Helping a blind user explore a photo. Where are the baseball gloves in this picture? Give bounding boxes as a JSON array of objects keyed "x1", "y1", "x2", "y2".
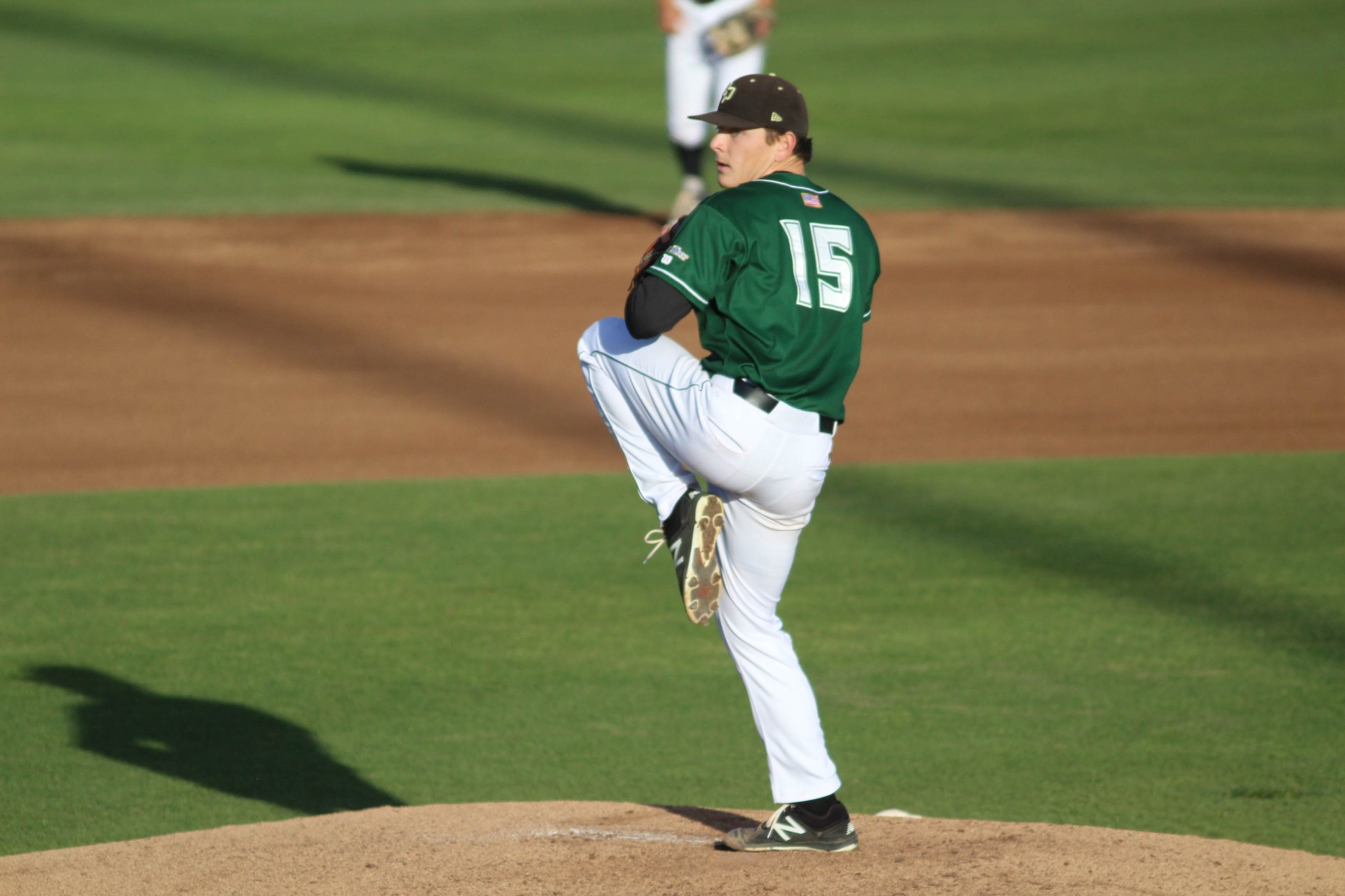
[{"x1": 704, "y1": 4, "x2": 777, "y2": 56}]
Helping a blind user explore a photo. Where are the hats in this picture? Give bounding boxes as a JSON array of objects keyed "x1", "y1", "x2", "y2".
[{"x1": 689, "y1": 72, "x2": 808, "y2": 140}]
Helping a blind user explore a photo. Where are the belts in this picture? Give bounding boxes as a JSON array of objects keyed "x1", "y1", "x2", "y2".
[{"x1": 732, "y1": 378, "x2": 834, "y2": 434}]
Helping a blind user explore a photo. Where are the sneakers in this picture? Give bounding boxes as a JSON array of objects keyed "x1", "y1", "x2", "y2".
[
  {"x1": 721, "y1": 802, "x2": 858, "y2": 853},
  {"x1": 663, "y1": 478, "x2": 724, "y2": 625}
]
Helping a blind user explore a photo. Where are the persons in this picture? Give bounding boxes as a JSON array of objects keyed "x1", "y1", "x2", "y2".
[
  {"x1": 660, "y1": 0, "x2": 773, "y2": 236},
  {"x1": 575, "y1": 73, "x2": 880, "y2": 854}
]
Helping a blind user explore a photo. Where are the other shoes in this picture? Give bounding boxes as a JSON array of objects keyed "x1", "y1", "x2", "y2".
[{"x1": 672, "y1": 176, "x2": 704, "y2": 217}]
